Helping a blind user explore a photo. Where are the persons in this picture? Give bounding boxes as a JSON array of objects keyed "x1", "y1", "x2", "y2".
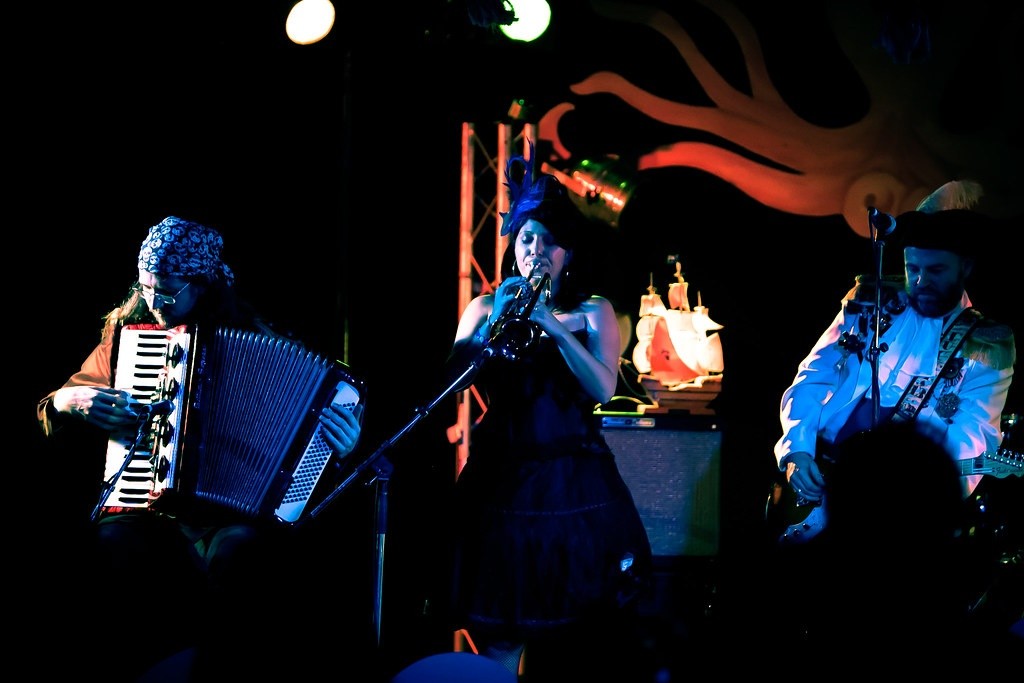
[
  {"x1": 32, "y1": 214, "x2": 364, "y2": 634},
  {"x1": 424, "y1": 176, "x2": 671, "y2": 683},
  {"x1": 668, "y1": 416, "x2": 1024, "y2": 683},
  {"x1": 772, "y1": 206, "x2": 1018, "y2": 548}
]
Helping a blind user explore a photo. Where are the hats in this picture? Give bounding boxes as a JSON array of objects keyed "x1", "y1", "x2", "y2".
[{"x1": 892, "y1": 178, "x2": 983, "y2": 263}]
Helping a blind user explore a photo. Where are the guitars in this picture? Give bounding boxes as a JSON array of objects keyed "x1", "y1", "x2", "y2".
[{"x1": 769, "y1": 427, "x2": 1024, "y2": 550}]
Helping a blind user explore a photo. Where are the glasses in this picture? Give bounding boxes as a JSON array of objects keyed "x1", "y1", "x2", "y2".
[{"x1": 132, "y1": 282, "x2": 191, "y2": 305}]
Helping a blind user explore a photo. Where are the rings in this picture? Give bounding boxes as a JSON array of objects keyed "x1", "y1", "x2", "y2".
[{"x1": 795, "y1": 489, "x2": 801, "y2": 495}]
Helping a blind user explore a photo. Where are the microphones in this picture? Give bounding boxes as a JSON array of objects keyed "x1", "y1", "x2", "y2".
[
  {"x1": 480, "y1": 327, "x2": 514, "y2": 359},
  {"x1": 867, "y1": 207, "x2": 897, "y2": 236},
  {"x1": 141, "y1": 400, "x2": 176, "y2": 415}
]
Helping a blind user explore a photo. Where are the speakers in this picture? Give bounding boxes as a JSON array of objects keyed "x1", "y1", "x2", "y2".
[{"x1": 595, "y1": 413, "x2": 730, "y2": 561}]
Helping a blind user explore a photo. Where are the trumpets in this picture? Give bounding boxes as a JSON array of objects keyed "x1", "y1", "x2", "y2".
[{"x1": 489, "y1": 258, "x2": 552, "y2": 362}]
